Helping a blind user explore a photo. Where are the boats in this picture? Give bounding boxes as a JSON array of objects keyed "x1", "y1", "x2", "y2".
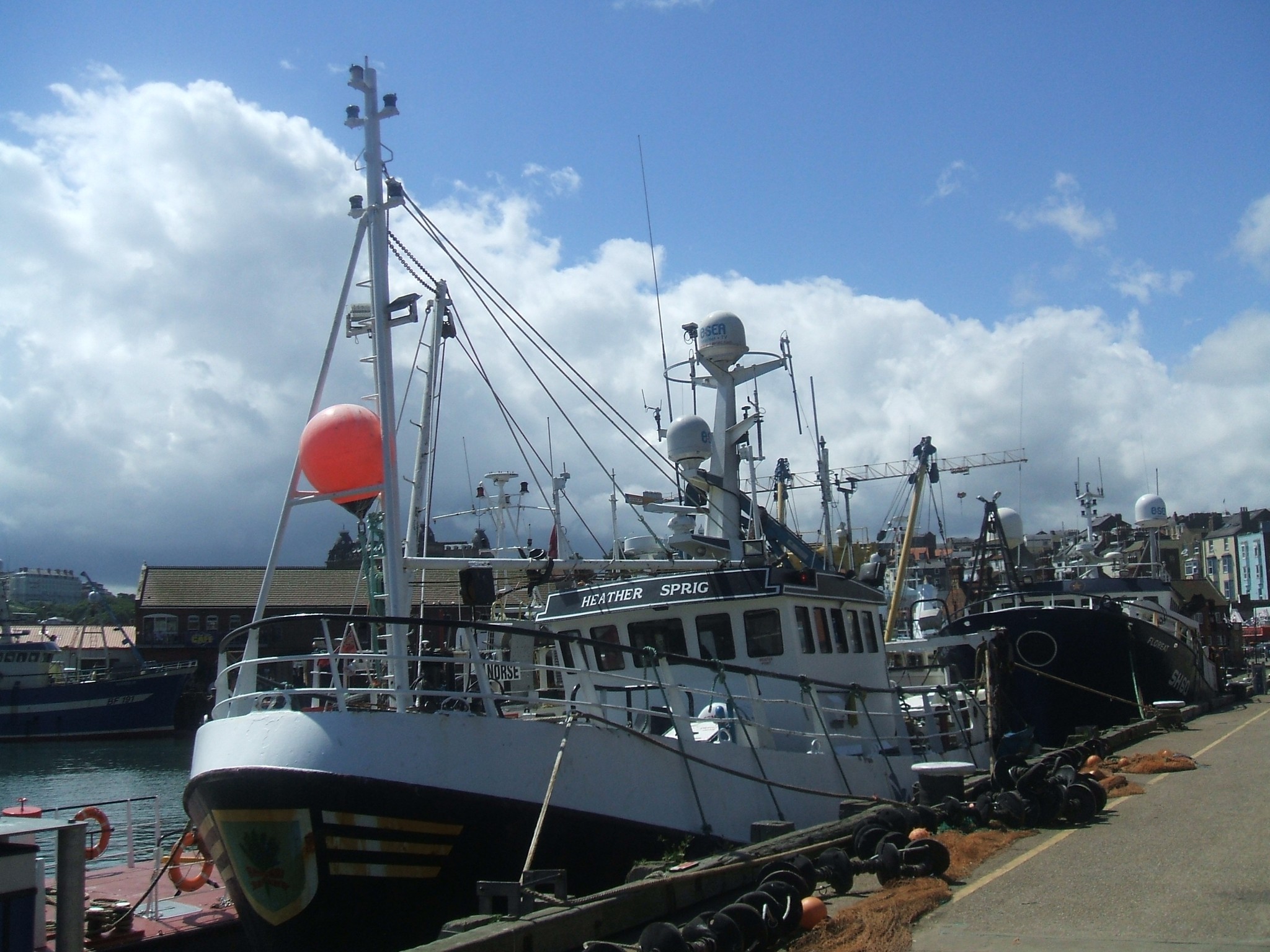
[
  {"x1": 180, "y1": 50, "x2": 1269, "y2": 952},
  {"x1": 0, "y1": 567, "x2": 200, "y2": 746}
]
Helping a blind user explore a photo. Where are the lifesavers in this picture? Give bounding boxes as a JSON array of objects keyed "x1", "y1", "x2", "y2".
[
  {"x1": 73, "y1": 806, "x2": 111, "y2": 859},
  {"x1": 170, "y1": 834, "x2": 213, "y2": 893}
]
